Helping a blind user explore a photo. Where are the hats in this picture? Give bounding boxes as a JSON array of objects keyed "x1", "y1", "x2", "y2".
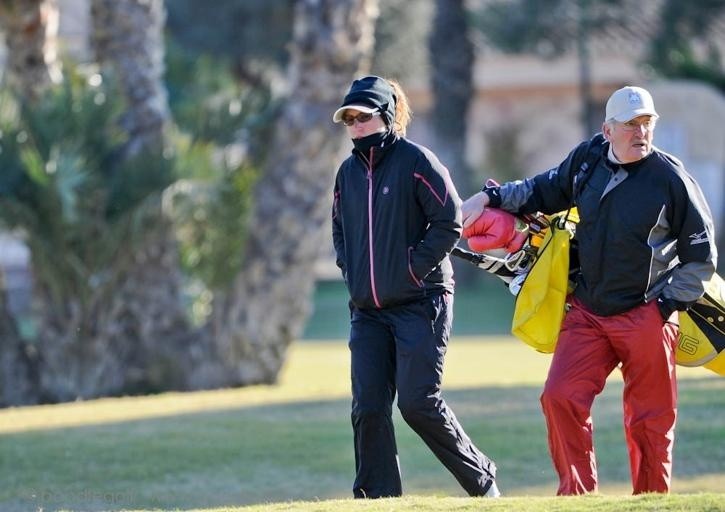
[
  {"x1": 333, "y1": 76, "x2": 395, "y2": 126},
  {"x1": 605, "y1": 86, "x2": 658, "y2": 124}
]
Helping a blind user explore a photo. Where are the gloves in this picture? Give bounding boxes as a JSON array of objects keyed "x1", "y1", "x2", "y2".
[{"x1": 461, "y1": 192, "x2": 490, "y2": 228}]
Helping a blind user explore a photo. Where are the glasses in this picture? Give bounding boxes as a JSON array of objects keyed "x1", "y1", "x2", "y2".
[
  {"x1": 342, "y1": 112, "x2": 381, "y2": 125},
  {"x1": 612, "y1": 121, "x2": 655, "y2": 130}
]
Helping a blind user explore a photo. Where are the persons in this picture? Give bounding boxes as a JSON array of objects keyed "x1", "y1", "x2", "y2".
[
  {"x1": 331, "y1": 76, "x2": 502, "y2": 501},
  {"x1": 462, "y1": 85, "x2": 720, "y2": 495}
]
